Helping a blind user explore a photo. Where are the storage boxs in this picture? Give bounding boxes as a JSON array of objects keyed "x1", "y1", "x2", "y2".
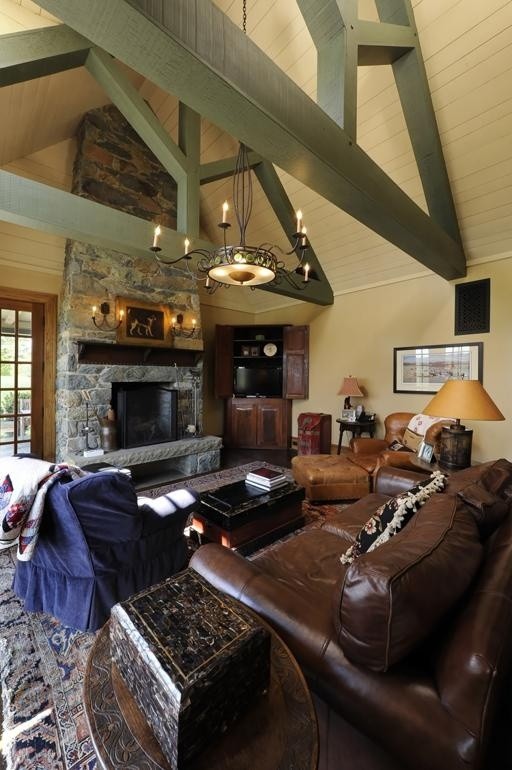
[{"x1": 110, "y1": 566, "x2": 272, "y2": 770}]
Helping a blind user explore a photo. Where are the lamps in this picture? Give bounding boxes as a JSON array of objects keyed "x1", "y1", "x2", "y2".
[
  {"x1": 171, "y1": 314, "x2": 196, "y2": 338},
  {"x1": 422, "y1": 380, "x2": 505, "y2": 470},
  {"x1": 91, "y1": 302, "x2": 124, "y2": 332},
  {"x1": 337, "y1": 375, "x2": 364, "y2": 409},
  {"x1": 150, "y1": 0, "x2": 311, "y2": 295}
]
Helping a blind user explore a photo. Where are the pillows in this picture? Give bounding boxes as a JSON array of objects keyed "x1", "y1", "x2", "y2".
[{"x1": 333, "y1": 459, "x2": 512, "y2": 675}]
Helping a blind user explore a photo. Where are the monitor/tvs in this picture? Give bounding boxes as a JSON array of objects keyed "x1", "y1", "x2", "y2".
[{"x1": 233, "y1": 363, "x2": 282, "y2": 398}]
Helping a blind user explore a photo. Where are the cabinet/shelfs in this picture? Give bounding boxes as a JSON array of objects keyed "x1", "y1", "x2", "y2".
[{"x1": 213, "y1": 323, "x2": 309, "y2": 450}]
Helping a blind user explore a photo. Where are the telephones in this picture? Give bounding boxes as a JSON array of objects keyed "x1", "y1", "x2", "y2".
[{"x1": 358, "y1": 411, "x2": 376, "y2": 422}]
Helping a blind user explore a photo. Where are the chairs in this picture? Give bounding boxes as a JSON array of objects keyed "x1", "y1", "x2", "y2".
[
  {"x1": 347, "y1": 412, "x2": 457, "y2": 490},
  {"x1": 11, "y1": 453, "x2": 201, "y2": 632}
]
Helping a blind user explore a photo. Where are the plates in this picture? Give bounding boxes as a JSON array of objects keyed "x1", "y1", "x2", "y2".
[{"x1": 263, "y1": 344, "x2": 278, "y2": 358}]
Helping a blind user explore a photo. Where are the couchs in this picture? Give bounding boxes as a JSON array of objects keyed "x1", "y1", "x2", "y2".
[{"x1": 188, "y1": 459, "x2": 512, "y2": 770}]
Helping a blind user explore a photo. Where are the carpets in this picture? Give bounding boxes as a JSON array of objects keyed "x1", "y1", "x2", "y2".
[{"x1": 0, "y1": 460, "x2": 360, "y2": 770}]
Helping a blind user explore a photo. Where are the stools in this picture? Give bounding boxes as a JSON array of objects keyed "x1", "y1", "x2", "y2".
[{"x1": 291, "y1": 454, "x2": 369, "y2": 510}]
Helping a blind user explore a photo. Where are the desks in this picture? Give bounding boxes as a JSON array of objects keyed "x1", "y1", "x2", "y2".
[
  {"x1": 84, "y1": 590, "x2": 320, "y2": 770},
  {"x1": 409, "y1": 456, "x2": 482, "y2": 477},
  {"x1": 336, "y1": 418, "x2": 377, "y2": 455}
]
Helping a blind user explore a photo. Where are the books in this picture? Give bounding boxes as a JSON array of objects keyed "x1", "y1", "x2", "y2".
[{"x1": 244, "y1": 467, "x2": 289, "y2": 492}]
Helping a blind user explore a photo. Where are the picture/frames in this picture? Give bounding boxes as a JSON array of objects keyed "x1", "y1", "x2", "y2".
[
  {"x1": 341, "y1": 409, "x2": 355, "y2": 420},
  {"x1": 418, "y1": 441, "x2": 436, "y2": 464},
  {"x1": 394, "y1": 342, "x2": 483, "y2": 395}
]
